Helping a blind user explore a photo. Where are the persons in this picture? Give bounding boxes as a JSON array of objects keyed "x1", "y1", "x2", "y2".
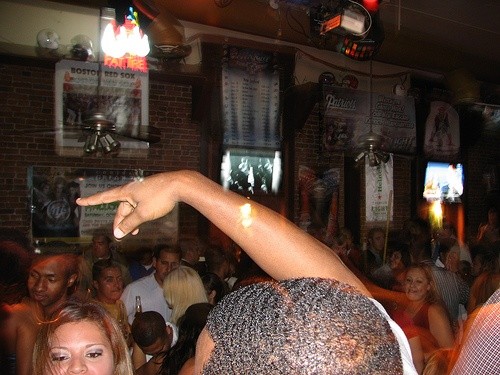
[
  {"x1": 0, "y1": 210, "x2": 500, "y2": 375},
  {"x1": 37, "y1": 178, "x2": 81, "y2": 222},
  {"x1": 76, "y1": 169, "x2": 419, "y2": 375},
  {"x1": 228, "y1": 159, "x2": 273, "y2": 194}
]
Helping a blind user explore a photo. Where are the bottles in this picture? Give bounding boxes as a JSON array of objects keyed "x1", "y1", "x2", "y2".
[{"x1": 136, "y1": 296, "x2": 142, "y2": 312}]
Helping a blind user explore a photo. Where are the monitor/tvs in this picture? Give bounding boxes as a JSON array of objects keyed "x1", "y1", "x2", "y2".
[
  {"x1": 420, "y1": 159, "x2": 467, "y2": 204},
  {"x1": 219, "y1": 146, "x2": 284, "y2": 197}
]
